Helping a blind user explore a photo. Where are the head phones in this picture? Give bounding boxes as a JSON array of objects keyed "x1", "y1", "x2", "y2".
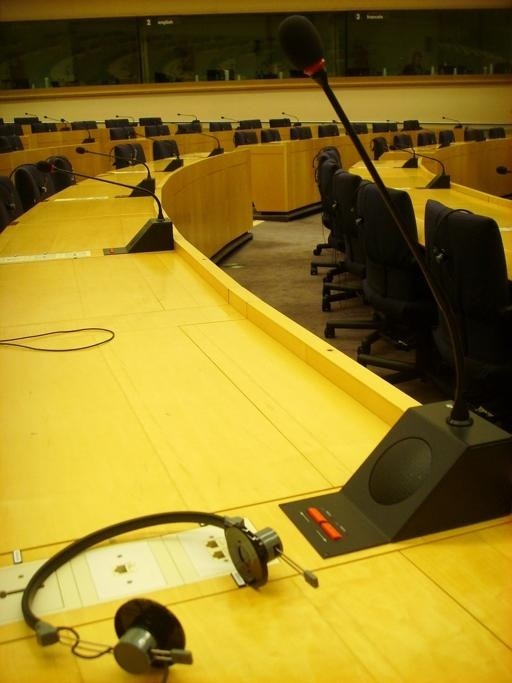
[
  {"x1": 313, "y1": 148, "x2": 345, "y2": 220},
  {"x1": 424, "y1": 208, "x2": 473, "y2": 277},
  {"x1": 22, "y1": 511, "x2": 321, "y2": 676},
  {"x1": 5, "y1": 164, "x2": 48, "y2": 210},
  {"x1": 349, "y1": 182, "x2": 375, "y2": 240}
]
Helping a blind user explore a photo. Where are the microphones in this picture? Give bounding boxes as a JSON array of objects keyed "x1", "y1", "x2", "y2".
[
  {"x1": 176, "y1": 112, "x2": 200, "y2": 126},
  {"x1": 333, "y1": 119, "x2": 338, "y2": 124},
  {"x1": 442, "y1": 116, "x2": 462, "y2": 129},
  {"x1": 130, "y1": 130, "x2": 160, "y2": 147},
  {"x1": 36, "y1": 161, "x2": 175, "y2": 255},
  {"x1": 115, "y1": 114, "x2": 137, "y2": 126},
  {"x1": 25, "y1": 112, "x2": 42, "y2": 123},
  {"x1": 44, "y1": 116, "x2": 70, "y2": 131},
  {"x1": 222, "y1": 116, "x2": 241, "y2": 130},
  {"x1": 61, "y1": 118, "x2": 95, "y2": 144},
  {"x1": 197, "y1": 129, "x2": 224, "y2": 157},
  {"x1": 389, "y1": 145, "x2": 450, "y2": 189},
  {"x1": 272, "y1": 15, "x2": 512, "y2": 562},
  {"x1": 282, "y1": 113, "x2": 299, "y2": 122},
  {"x1": 496, "y1": 166, "x2": 512, "y2": 175},
  {"x1": 75, "y1": 147, "x2": 156, "y2": 198}
]
